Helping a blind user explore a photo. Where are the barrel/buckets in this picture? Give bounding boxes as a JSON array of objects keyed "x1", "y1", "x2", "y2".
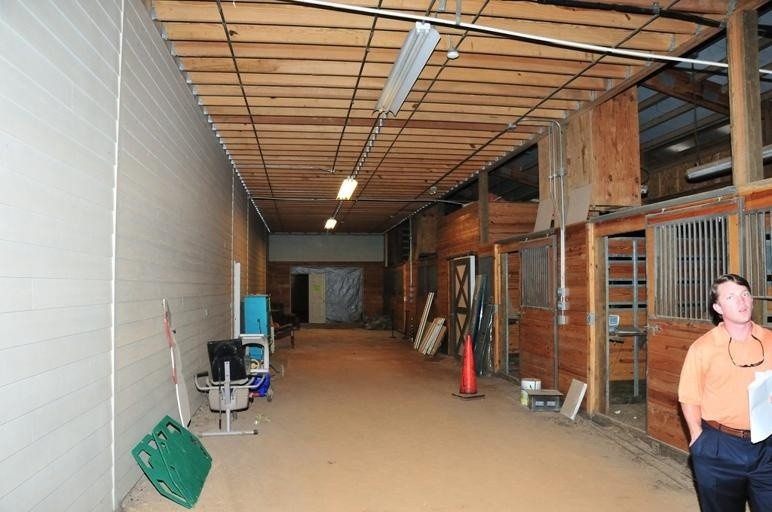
[{"x1": 521, "y1": 378, "x2": 542, "y2": 406}]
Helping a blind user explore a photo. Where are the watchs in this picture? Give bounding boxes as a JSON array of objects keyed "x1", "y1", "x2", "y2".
[{"x1": 672, "y1": 271, "x2": 772, "y2": 511}]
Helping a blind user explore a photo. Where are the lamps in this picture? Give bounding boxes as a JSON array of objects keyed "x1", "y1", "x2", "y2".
[
  {"x1": 336, "y1": 179, "x2": 358, "y2": 201},
  {"x1": 324, "y1": 218, "x2": 337, "y2": 230},
  {"x1": 684, "y1": 53, "x2": 772, "y2": 180},
  {"x1": 371, "y1": 23, "x2": 441, "y2": 116}
]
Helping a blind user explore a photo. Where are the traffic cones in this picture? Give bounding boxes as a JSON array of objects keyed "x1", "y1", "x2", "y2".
[{"x1": 451, "y1": 333, "x2": 484, "y2": 399}]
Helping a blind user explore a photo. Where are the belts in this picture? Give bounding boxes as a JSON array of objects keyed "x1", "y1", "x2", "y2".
[{"x1": 704, "y1": 419, "x2": 751, "y2": 441}]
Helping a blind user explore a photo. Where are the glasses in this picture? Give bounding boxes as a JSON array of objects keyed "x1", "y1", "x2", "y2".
[{"x1": 721, "y1": 334, "x2": 768, "y2": 372}]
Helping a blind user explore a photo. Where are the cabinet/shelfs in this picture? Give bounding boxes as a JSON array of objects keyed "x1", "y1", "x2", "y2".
[{"x1": 244, "y1": 296, "x2": 269, "y2": 362}]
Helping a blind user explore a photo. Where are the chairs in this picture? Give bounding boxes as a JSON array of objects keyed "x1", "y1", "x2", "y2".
[{"x1": 270, "y1": 303, "x2": 300, "y2": 349}]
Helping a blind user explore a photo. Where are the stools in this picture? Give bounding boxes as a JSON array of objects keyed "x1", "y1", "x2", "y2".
[{"x1": 529, "y1": 390, "x2": 563, "y2": 411}]
